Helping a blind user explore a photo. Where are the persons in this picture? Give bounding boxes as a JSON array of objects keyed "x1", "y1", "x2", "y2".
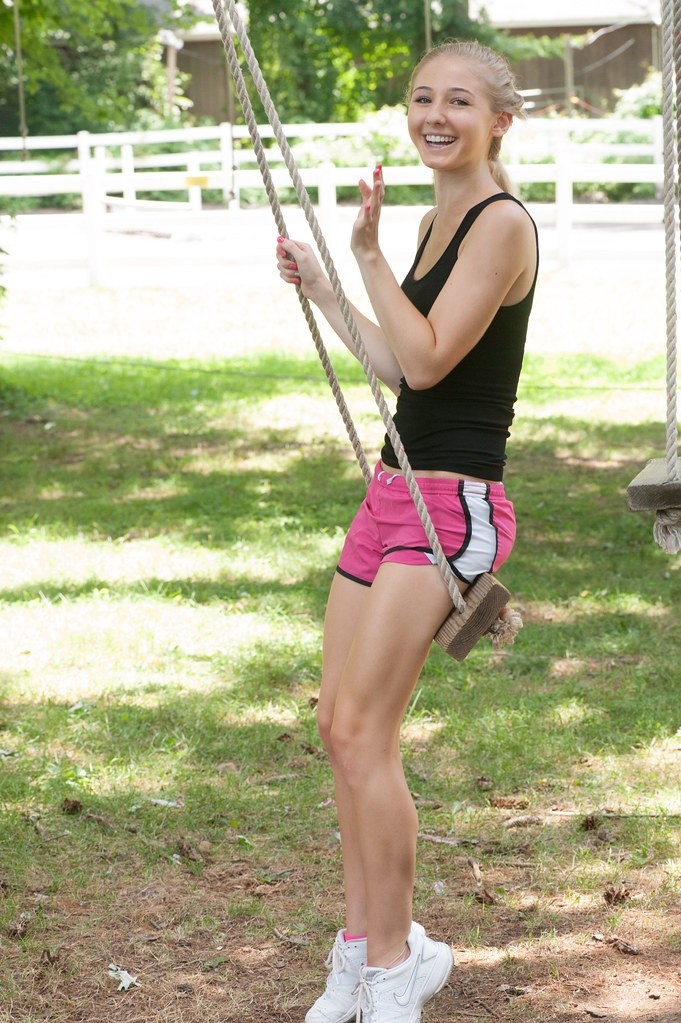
[{"x1": 277, "y1": 43, "x2": 538, "y2": 1023}]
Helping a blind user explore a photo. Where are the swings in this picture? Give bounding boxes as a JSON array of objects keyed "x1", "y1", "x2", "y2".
[
  {"x1": 214, "y1": 1, "x2": 513, "y2": 665},
  {"x1": 622, "y1": 2, "x2": 680, "y2": 514}
]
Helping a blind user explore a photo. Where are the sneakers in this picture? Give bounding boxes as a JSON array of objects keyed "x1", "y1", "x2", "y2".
[
  {"x1": 305, "y1": 922, "x2": 429, "y2": 1023},
  {"x1": 351, "y1": 929, "x2": 456, "y2": 1023}
]
